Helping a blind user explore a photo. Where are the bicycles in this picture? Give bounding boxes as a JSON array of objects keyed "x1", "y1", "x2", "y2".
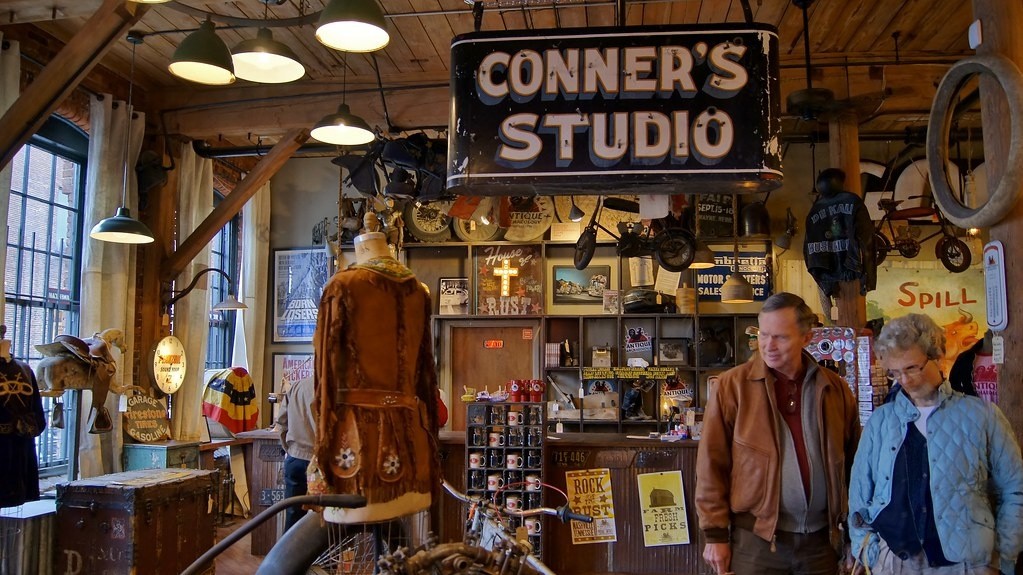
[
  {"x1": 574, "y1": 194, "x2": 698, "y2": 272},
  {"x1": 870, "y1": 192, "x2": 972, "y2": 273},
  {"x1": 176, "y1": 478, "x2": 594, "y2": 575}
]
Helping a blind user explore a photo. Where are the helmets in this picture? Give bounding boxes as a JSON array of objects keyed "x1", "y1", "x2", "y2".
[{"x1": 816, "y1": 167, "x2": 846, "y2": 199}]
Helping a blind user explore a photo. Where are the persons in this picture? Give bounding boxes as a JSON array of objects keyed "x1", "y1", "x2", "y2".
[
  {"x1": 846, "y1": 313, "x2": 1023, "y2": 575},
  {"x1": 694, "y1": 291, "x2": 861, "y2": 575},
  {"x1": 949, "y1": 329, "x2": 998, "y2": 407},
  {"x1": 0, "y1": 338, "x2": 46, "y2": 507},
  {"x1": 275, "y1": 372, "x2": 317, "y2": 535},
  {"x1": 306, "y1": 232, "x2": 444, "y2": 525}
]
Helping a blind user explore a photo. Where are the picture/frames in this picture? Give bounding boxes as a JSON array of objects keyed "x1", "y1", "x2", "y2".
[
  {"x1": 438, "y1": 277, "x2": 468, "y2": 315},
  {"x1": 651, "y1": 337, "x2": 691, "y2": 367},
  {"x1": 553, "y1": 265, "x2": 611, "y2": 305},
  {"x1": 270, "y1": 247, "x2": 334, "y2": 428}
]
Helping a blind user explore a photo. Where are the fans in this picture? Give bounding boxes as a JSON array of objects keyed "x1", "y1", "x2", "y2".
[{"x1": 779, "y1": 0, "x2": 913, "y2": 122}]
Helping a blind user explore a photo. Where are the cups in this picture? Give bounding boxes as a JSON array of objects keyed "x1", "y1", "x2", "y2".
[
  {"x1": 489, "y1": 432, "x2": 504, "y2": 447},
  {"x1": 506, "y1": 497, "x2": 522, "y2": 511},
  {"x1": 488, "y1": 476, "x2": 504, "y2": 490},
  {"x1": 525, "y1": 517, "x2": 542, "y2": 534},
  {"x1": 675, "y1": 288, "x2": 695, "y2": 314},
  {"x1": 469, "y1": 453, "x2": 485, "y2": 468},
  {"x1": 525, "y1": 476, "x2": 541, "y2": 490},
  {"x1": 507, "y1": 411, "x2": 523, "y2": 426},
  {"x1": 474, "y1": 434, "x2": 481, "y2": 446},
  {"x1": 506, "y1": 454, "x2": 522, "y2": 469}
]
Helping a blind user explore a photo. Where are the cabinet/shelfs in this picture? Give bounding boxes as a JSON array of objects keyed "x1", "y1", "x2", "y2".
[
  {"x1": 340, "y1": 238, "x2": 772, "y2": 564},
  {"x1": 121, "y1": 441, "x2": 199, "y2": 473}
]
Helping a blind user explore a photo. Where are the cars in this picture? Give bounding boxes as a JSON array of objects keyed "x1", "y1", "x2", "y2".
[{"x1": 441, "y1": 288, "x2": 469, "y2": 305}]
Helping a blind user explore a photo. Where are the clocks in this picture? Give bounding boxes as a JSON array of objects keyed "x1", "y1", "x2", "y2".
[{"x1": 147, "y1": 334, "x2": 187, "y2": 395}]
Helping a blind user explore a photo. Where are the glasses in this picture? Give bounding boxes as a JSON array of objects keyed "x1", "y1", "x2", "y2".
[
  {"x1": 786, "y1": 379, "x2": 797, "y2": 414},
  {"x1": 886, "y1": 356, "x2": 929, "y2": 381}
]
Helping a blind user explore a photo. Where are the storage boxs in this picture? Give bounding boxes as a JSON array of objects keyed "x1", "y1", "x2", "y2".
[{"x1": 54, "y1": 468, "x2": 219, "y2": 575}]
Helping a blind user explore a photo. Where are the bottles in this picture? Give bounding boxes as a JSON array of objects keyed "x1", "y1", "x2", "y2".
[
  {"x1": 509, "y1": 429, "x2": 517, "y2": 446},
  {"x1": 528, "y1": 451, "x2": 534, "y2": 468},
  {"x1": 530, "y1": 408, "x2": 536, "y2": 425},
  {"x1": 472, "y1": 471, "x2": 478, "y2": 489},
  {"x1": 508, "y1": 472, "x2": 516, "y2": 489},
  {"x1": 489, "y1": 493, "x2": 536, "y2": 510},
  {"x1": 528, "y1": 429, "x2": 536, "y2": 446},
  {"x1": 508, "y1": 514, "x2": 515, "y2": 532},
  {"x1": 491, "y1": 450, "x2": 497, "y2": 467},
  {"x1": 491, "y1": 407, "x2": 499, "y2": 424},
  {"x1": 529, "y1": 538, "x2": 534, "y2": 554},
  {"x1": 505, "y1": 380, "x2": 546, "y2": 402}
]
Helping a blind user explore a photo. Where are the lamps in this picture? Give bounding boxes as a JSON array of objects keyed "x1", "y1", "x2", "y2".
[
  {"x1": 230, "y1": 0, "x2": 305, "y2": 83},
  {"x1": 310, "y1": 52, "x2": 375, "y2": 145},
  {"x1": 687, "y1": 193, "x2": 716, "y2": 269},
  {"x1": 165, "y1": 13, "x2": 235, "y2": 85},
  {"x1": 89, "y1": 28, "x2": 154, "y2": 244},
  {"x1": 720, "y1": 194, "x2": 754, "y2": 304},
  {"x1": 773, "y1": 207, "x2": 797, "y2": 250},
  {"x1": 568, "y1": 194, "x2": 585, "y2": 222},
  {"x1": 162, "y1": 268, "x2": 249, "y2": 315},
  {"x1": 314, "y1": 0, "x2": 391, "y2": 53}
]
